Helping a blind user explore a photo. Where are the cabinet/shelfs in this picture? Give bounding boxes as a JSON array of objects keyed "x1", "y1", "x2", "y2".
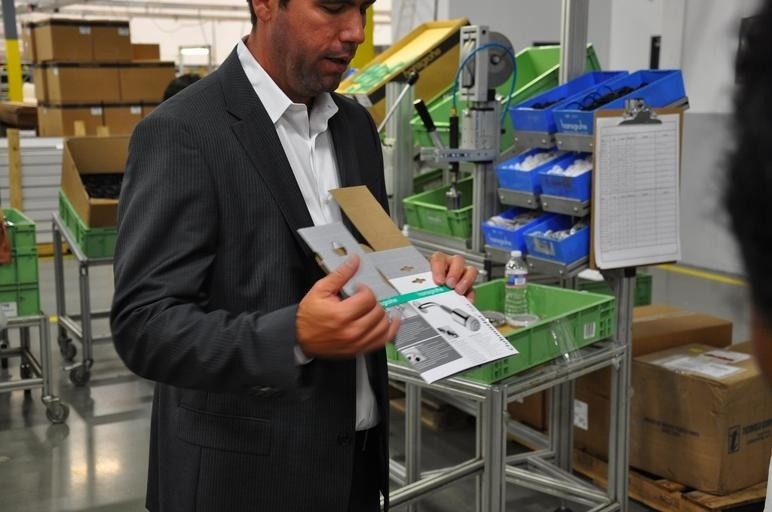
[{"x1": 340, "y1": 47, "x2": 637, "y2": 511}]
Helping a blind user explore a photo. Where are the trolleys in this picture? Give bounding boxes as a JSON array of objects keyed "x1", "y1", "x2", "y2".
[
  {"x1": 1, "y1": 313, "x2": 68, "y2": 424},
  {"x1": 51, "y1": 214, "x2": 115, "y2": 388}
]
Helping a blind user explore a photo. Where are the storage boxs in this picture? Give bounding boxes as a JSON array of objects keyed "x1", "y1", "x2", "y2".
[
  {"x1": 544, "y1": 303, "x2": 772, "y2": 495},
  {"x1": 20, "y1": 15, "x2": 180, "y2": 228}
]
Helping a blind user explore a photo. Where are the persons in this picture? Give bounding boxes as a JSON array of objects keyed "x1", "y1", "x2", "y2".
[
  {"x1": 158, "y1": 70, "x2": 201, "y2": 101},
  {"x1": 696, "y1": 1, "x2": 771, "y2": 387},
  {"x1": 106, "y1": 2, "x2": 482, "y2": 512}
]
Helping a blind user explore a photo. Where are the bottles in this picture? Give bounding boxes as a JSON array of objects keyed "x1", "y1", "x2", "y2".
[{"x1": 506, "y1": 252, "x2": 529, "y2": 322}]
